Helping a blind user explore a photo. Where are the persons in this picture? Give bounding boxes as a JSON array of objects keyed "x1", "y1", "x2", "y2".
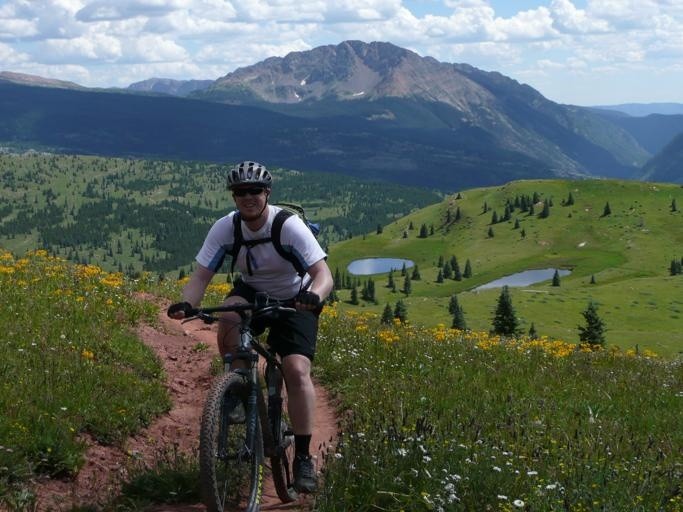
[{"x1": 164, "y1": 159, "x2": 336, "y2": 496}]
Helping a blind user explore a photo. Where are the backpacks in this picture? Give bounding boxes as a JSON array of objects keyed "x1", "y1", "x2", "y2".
[{"x1": 227, "y1": 202, "x2": 320, "y2": 288}]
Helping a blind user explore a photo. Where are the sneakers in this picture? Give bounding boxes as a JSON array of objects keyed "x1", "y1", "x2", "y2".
[
  {"x1": 292, "y1": 455, "x2": 318, "y2": 494},
  {"x1": 227, "y1": 392, "x2": 248, "y2": 424}
]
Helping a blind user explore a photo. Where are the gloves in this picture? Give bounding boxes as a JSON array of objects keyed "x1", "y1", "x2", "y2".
[
  {"x1": 167, "y1": 303, "x2": 194, "y2": 317},
  {"x1": 294, "y1": 289, "x2": 320, "y2": 305}
]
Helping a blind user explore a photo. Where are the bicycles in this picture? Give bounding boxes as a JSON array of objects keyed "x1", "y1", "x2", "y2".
[{"x1": 170, "y1": 294, "x2": 297, "y2": 512}]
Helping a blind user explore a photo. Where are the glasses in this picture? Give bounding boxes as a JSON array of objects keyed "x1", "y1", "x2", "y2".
[{"x1": 233, "y1": 188, "x2": 264, "y2": 197}]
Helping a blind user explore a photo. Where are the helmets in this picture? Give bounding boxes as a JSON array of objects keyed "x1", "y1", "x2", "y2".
[{"x1": 227, "y1": 161, "x2": 273, "y2": 188}]
